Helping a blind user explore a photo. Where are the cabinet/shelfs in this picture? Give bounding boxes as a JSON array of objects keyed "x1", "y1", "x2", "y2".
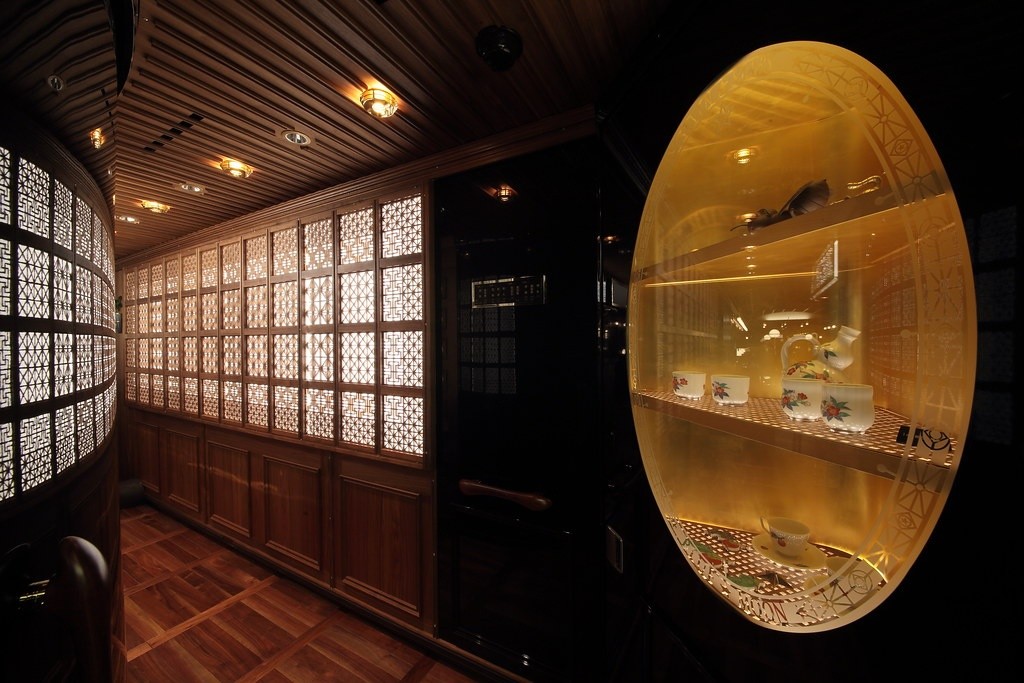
[{"x1": 628, "y1": 165, "x2": 960, "y2": 630}]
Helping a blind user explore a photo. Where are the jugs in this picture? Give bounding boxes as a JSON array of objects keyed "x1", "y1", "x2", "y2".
[{"x1": 780, "y1": 325, "x2": 861, "y2": 384}]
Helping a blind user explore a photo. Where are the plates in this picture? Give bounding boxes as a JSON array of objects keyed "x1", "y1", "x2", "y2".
[
  {"x1": 826, "y1": 557, "x2": 858, "y2": 581},
  {"x1": 752, "y1": 533, "x2": 827, "y2": 569}
]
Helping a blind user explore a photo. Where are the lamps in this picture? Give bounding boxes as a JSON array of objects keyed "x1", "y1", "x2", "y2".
[
  {"x1": 141, "y1": 199, "x2": 170, "y2": 215},
  {"x1": 358, "y1": 85, "x2": 401, "y2": 124},
  {"x1": 215, "y1": 154, "x2": 257, "y2": 183},
  {"x1": 89, "y1": 127, "x2": 104, "y2": 150},
  {"x1": 492, "y1": 183, "x2": 522, "y2": 206}
]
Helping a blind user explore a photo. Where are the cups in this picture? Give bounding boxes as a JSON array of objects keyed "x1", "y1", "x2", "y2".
[
  {"x1": 671, "y1": 370, "x2": 707, "y2": 401},
  {"x1": 760, "y1": 515, "x2": 810, "y2": 557},
  {"x1": 780, "y1": 378, "x2": 826, "y2": 422},
  {"x1": 821, "y1": 382, "x2": 876, "y2": 435},
  {"x1": 710, "y1": 374, "x2": 750, "y2": 407}
]
